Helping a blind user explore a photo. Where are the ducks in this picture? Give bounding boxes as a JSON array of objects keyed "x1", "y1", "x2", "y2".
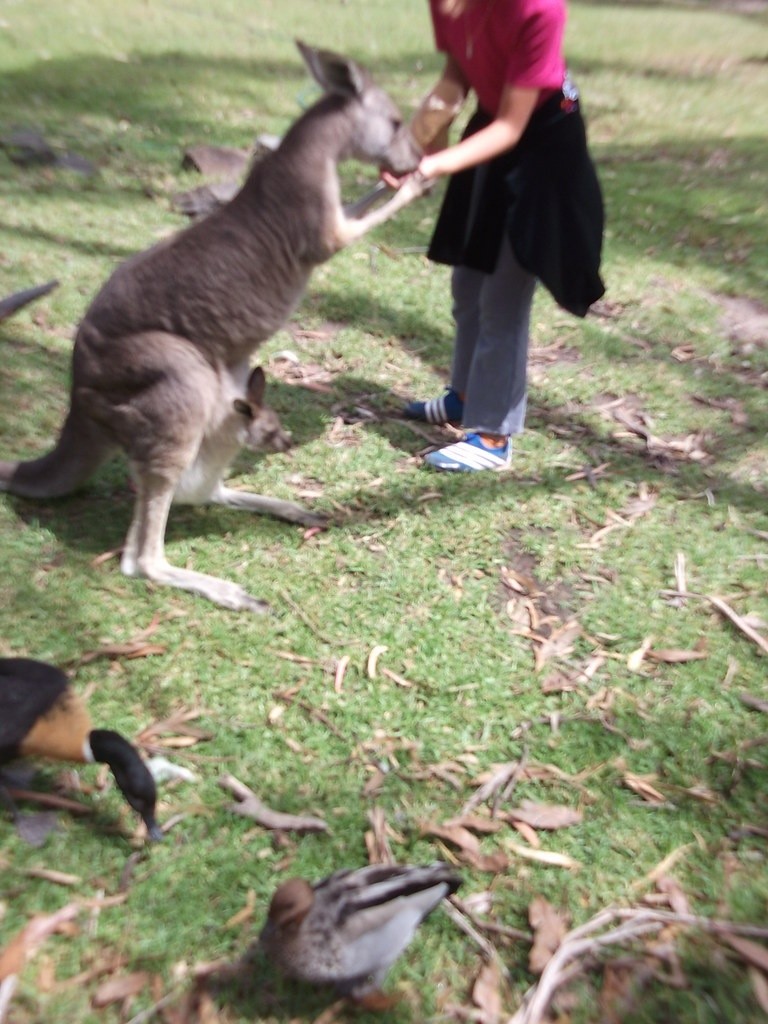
[
  {"x1": 1, "y1": 653, "x2": 172, "y2": 847},
  {"x1": 250, "y1": 857, "x2": 468, "y2": 1015}
]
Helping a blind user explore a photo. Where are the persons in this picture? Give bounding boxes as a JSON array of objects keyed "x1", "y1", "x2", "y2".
[{"x1": 382, "y1": 0, "x2": 607, "y2": 471}]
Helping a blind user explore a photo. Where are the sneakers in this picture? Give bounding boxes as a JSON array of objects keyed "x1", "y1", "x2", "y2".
[
  {"x1": 425, "y1": 433, "x2": 511, "y2": 471},
  {"x1": 405, "y1": 390, "x2": 465, "y2": 424}
]
